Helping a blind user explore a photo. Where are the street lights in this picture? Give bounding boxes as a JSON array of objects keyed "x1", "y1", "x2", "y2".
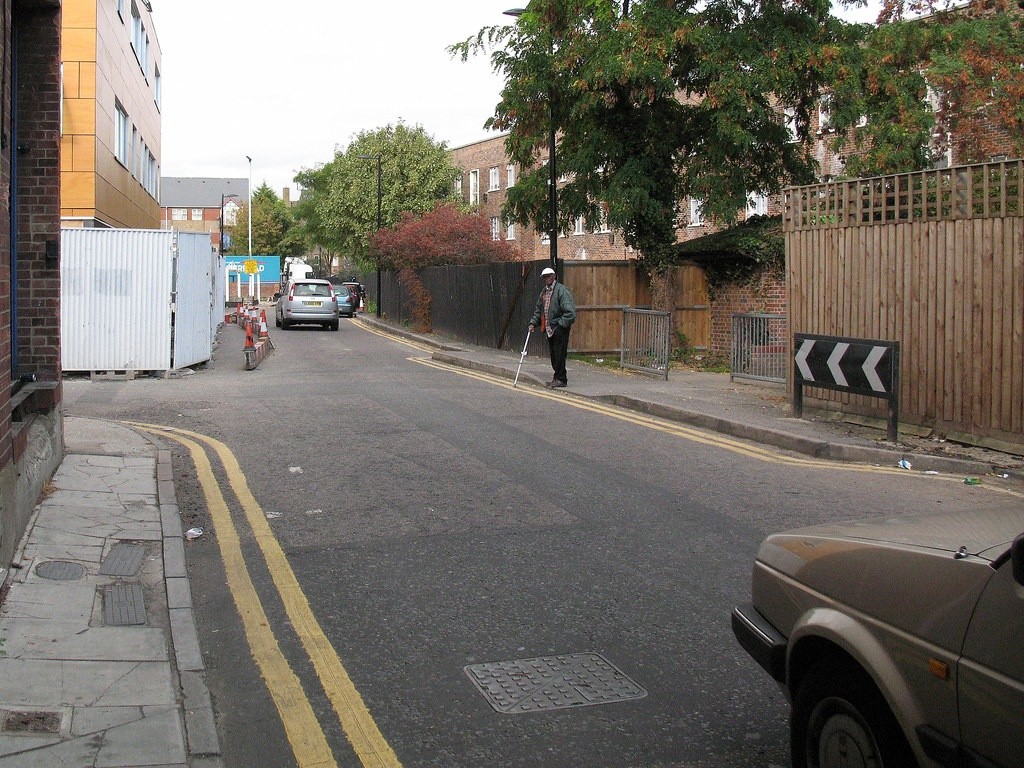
[
  {"x1": 219, "y1": 193, "x2": 239, "y2": 257},
  {"x1": 503, "y1": 7, "x2": 558, "y2": 288},
  {"x1": 243, "y1": 154, "x2": 258, "y2": 300},
  {"x1": 358, "y1": 153, "x2": 383, "y2": 319}
]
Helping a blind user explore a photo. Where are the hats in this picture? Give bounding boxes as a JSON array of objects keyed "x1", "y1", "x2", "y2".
[{"x1": 540, "y1": 268, "x2": 555, "y2": 277}]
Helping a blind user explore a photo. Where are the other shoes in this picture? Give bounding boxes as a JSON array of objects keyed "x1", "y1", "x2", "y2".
[{"x1": 547, "y1": 378, "x2": 566, "y2": 386}]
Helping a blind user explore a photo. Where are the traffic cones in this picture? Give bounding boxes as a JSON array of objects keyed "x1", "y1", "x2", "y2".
[
  {"x1": 358, "y1": 297, "x2": 364, "y2": 311},
  {"x1": 243, "y1": 321, "x2": 257, "y2": 352},
  {"x1": 238, "y1": 302, "x2": 268, "y2": 338}
]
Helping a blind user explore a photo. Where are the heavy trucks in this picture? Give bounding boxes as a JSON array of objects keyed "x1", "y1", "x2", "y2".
[{"x1": 225, "y1": 255, "x2": 313, "y2": 303}]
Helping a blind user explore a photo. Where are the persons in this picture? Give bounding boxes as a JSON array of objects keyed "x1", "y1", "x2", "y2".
[{"x1": 528, "y1": 267, "x2": 576, "y2": 388}]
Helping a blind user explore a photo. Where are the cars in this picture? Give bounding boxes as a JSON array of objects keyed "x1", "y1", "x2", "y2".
[
  {"x1": 273, "y1": 278, "x2": 340, "y2": 331},
  {"x1": 322, "y1": 282, "x2": 362, "y2": 318},
  {"x1": 730, "y1": 500, "x2": 1023, "y2": 768}
]
